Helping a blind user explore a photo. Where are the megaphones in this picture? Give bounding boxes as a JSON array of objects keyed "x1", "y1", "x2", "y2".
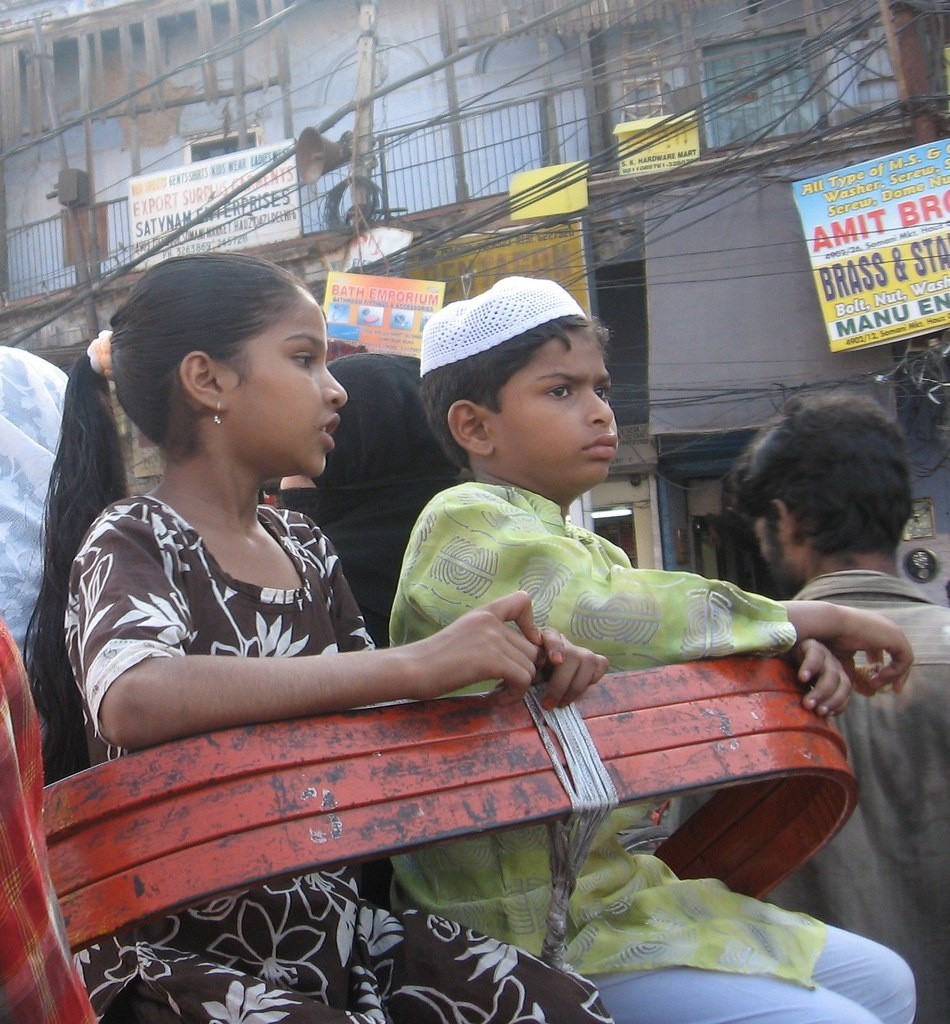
[{"x1": 295, "y1": 126, "x2": 351, "y2": 185}]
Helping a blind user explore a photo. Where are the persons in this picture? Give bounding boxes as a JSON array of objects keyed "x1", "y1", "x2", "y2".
[
  {"x1": 20, "y1": 253, "x2": 613, "y2": 1023},
  {"x1": 731, "y1": 388, "x2": 950, "y2": 1024},
  {"x1": 280, "y1": 274, "x2": 920, "y2": 1023}
]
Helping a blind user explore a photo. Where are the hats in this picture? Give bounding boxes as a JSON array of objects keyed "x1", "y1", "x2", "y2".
[{"x1": 417, "y1": 277, "x2": 593, "y2": 377}]
